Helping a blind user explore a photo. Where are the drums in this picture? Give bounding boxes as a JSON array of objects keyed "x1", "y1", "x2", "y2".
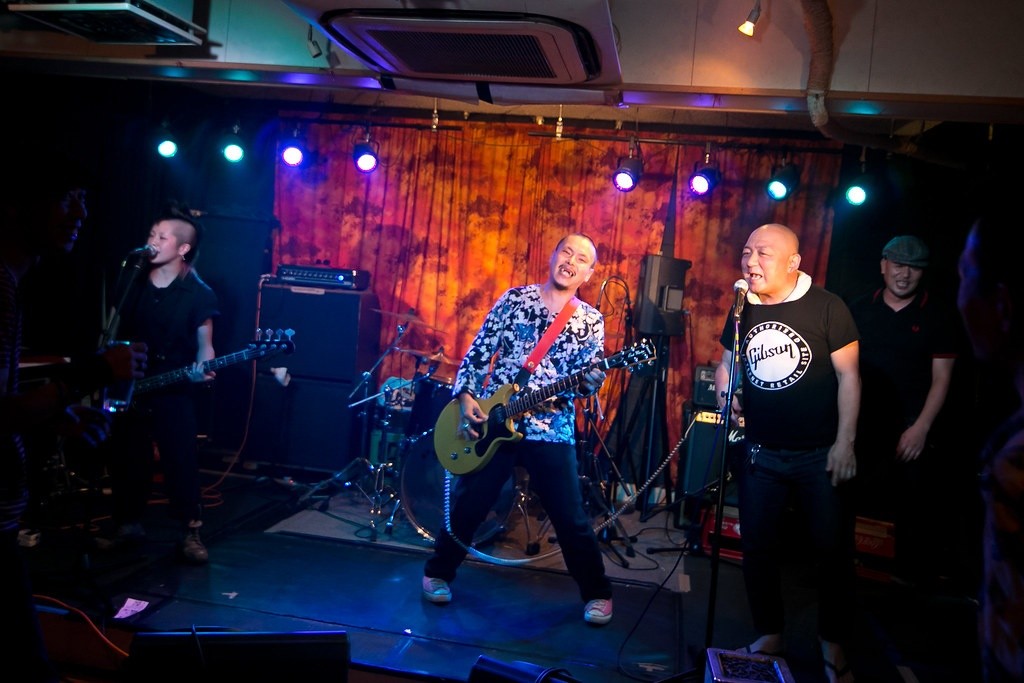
[
  {"x1": 403, "y1": 373, "x2": 456, "y2": 443},
  {"x1": 398, "y1": 428, "x2": 519, "y2": 547}
]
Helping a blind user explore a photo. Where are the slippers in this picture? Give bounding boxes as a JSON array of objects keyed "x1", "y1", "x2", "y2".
[
  {"x1": 734, "y1": 643, "x2": 792, "y2": 658},
  {"x1": 822, "y1": 657, "x2": 855, "y2": 683}
]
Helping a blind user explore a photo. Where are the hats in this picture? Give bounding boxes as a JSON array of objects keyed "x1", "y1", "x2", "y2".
[{"x1": 882, "y1": 236, "x2": 930, "y2": 267}]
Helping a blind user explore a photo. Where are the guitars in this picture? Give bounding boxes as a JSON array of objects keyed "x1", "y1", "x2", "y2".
[
  {"x1": 434, "y1": 338, "x2": 659, "y2": 476},
  {"x1": 82, "y1": 326, "x2": 298, "y2": 416}
]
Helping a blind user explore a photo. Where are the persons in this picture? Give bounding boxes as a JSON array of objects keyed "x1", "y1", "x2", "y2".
[
  {"x1": 110, "y1": 218, "x2": 221, "y2": 564},
  {"x1": 422, "y1": 233, "x2": 613, "y2": 624},
  {"x1": 958, "y1": 218, "x2": 1024, "y2": 683},
  {"x1": 0, "y1": 171, "x2": 89, "y2": 683},
  {"x1": 714, "y1": 222, "x2": 862, "y2": 683},
  {"x1": 874, "y1": 236, "x2": 974, "y2": 566}
]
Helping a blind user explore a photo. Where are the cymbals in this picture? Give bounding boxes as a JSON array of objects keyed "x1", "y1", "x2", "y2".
[
  {"x1": 402, "y1": 348, "x2": 463, "y2": 366},
  {"x1": 370, "y1": 307, "x2": 449, "y2": 335}
]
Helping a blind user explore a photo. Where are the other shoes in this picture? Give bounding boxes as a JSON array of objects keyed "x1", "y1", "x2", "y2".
[
  {"x1": 583, "y1": 598, "x2": 613, "y2": 624},
  {"x1": 422, "y1": 575, "x2": 452, "y2": 601},
  {"x1": 182, "y1": 519, "x2": 210, "y2": 562}
]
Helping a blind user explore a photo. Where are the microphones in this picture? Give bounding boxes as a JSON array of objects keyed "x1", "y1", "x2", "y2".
[
  {"x1": 131, "y1": 245, "x2": 158, "y2": 258},
  {"x1": 733, "y1": 278, "x2": 749, "y2": 318}
]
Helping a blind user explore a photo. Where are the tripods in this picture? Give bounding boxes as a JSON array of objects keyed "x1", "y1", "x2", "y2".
[
  {"x1": 647, "y1": 317, "x2": 745, "y2": 569},
  {"x1": 532, "y1": 388, "x2": 639, "y2": 567},
  {"x1": 287, "y1": 321, "x2": 439, "y2": 540}
]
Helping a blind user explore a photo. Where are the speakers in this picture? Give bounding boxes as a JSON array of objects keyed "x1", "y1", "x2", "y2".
[
  {"x1": 678, "y1": 408, "x2": 746, "y2": 500},
  {"x1": 254, "y1": 282, "x2": 381, "y2": 382},
  {"x1": 119, "y1": 629, "x2": 352, "y2": 683},
  {"x1": 632, "y1": 254, "x2": 692, "y2": 335},
  {"x1": 174, "y1": 209, "x2": 275, "y2": 456},
  {"x1": 248, "y1": 374, "x2": 377, "y2": 474}
]
